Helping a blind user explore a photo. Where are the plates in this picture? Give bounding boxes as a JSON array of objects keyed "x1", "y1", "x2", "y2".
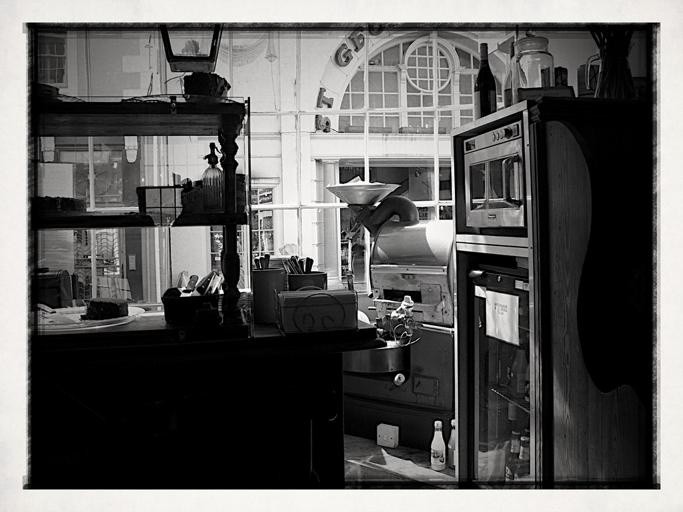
[{"x1": 37, "y1": 305, "x2": 146, "y2": 334}]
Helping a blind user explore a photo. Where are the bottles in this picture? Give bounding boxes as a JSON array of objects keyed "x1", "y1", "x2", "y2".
[
  {"x1": 513, "y1": 434, "x2": 530, "y2": 481},
  {"x1": 508, "y1": 344, "x2": 529, "y2": 400},
  {"x1": 503, "y1": 429, "x2": 522, "y2": 481},
  {"x1": 447, "y1": 419, "x2": 456, "y2": 469},
  {"x1": 430, "y1": 420, "x2": 445, "y2": 471}
]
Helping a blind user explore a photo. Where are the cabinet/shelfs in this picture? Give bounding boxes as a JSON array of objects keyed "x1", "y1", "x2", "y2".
[{"x1": 28, "y1": 99, "x2": 251, "y2": 335}]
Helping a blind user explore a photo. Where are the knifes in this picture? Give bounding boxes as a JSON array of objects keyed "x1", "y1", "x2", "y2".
[
  {"x1": 179, "y1": 275, "x2": 198, "y2": 298},
  {"x1": 37, "y1": 303, "x2": 76, "y2": 324}
]
